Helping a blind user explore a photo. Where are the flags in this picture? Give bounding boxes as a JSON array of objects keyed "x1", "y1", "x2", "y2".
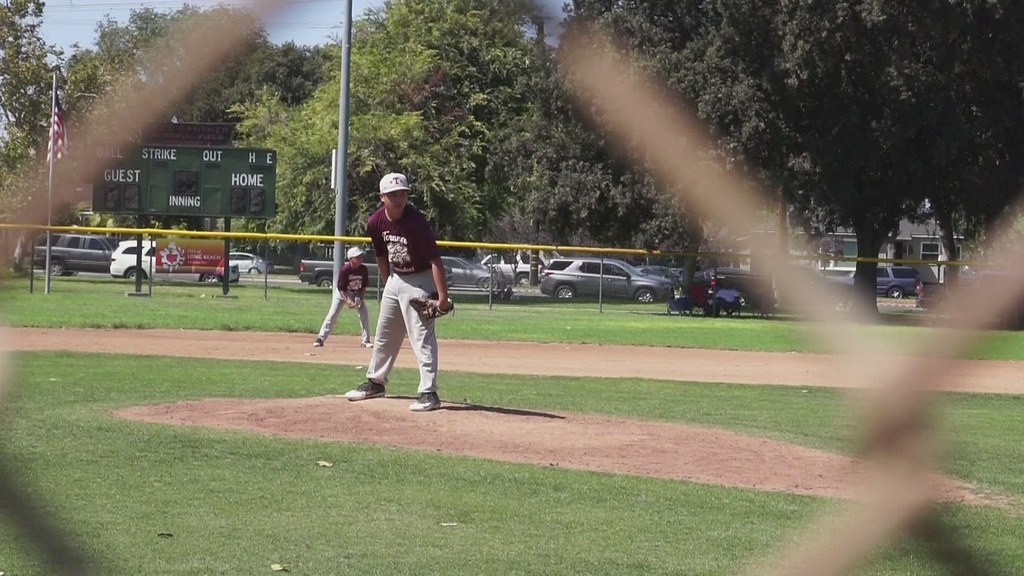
[{"x1": 45, "y1": 87, "x2": 68, "y2": 164}]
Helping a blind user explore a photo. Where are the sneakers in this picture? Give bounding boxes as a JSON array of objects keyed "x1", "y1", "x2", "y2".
[
  {"x1": 314, "y1": 339, "x2": 324, "y2": 347},
  {"x1": 361, "y1": 340, "x2": 374, "y2": 348},
  {"x1": 347, "y1": 380, "x2": 386, "y2": 400},
  {"x1": 409, "y1": 392, "x2": 441, "y2": 411}
]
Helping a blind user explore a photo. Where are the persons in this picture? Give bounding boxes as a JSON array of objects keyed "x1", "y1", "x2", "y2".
[
  {"x1": 673, "y1": 278, "x2": 687, "y2": 316},
  {"x1": 345, "y1": 172, "x2": 455, "y2": 411},
  {"x1": 313, "y1": 246, "x2": 374, "y2": 348}
]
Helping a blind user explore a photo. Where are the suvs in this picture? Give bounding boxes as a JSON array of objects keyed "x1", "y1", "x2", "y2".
[
  {"x1": 876, "y1": 265, "x2": 921, "y2": 299},
  {"x1": 34, "y1": 232, "x2": 122, "y2": 277},
  {"x1": 480, "y1": 253, "x2": 546, "y2": 285},
  {"x1": 541, "y1": 256, "x2": 676, "y2": 304},
  {"x1": 110, "y1": 240, "x2": 240, "y2": 284}
]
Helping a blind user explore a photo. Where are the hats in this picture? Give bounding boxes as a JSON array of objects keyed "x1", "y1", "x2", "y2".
[
  {"x1": 379, "y1": 173, "x2": 411, "y2": 194},
  {"x1": 348, "y1": 247, "x2": 362, "y2": 261}
]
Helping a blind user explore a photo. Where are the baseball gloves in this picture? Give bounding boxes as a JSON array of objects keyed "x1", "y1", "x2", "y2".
[
  {"x1": 346, "y1": 293, "x2": 362, "y2": 309},
  {"x1": 409, "y1": 292, "x2": 455, "y2": 319}
]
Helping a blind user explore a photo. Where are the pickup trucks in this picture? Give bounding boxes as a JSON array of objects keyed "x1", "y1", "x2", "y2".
[{"x1": 299, "y1": 248, "x2": 455, "y2": 290}]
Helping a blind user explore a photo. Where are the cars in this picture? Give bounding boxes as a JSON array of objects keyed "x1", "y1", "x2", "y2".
[
  {"x1": 229, "y1": 251, "x2": 274, "y2": 275},
  {"x1": 635, "y1": 265, "x2": 854, "y2": 313},
  {"x1": 441, "y1": 255, "x2": 507, "y2": 291}
]
